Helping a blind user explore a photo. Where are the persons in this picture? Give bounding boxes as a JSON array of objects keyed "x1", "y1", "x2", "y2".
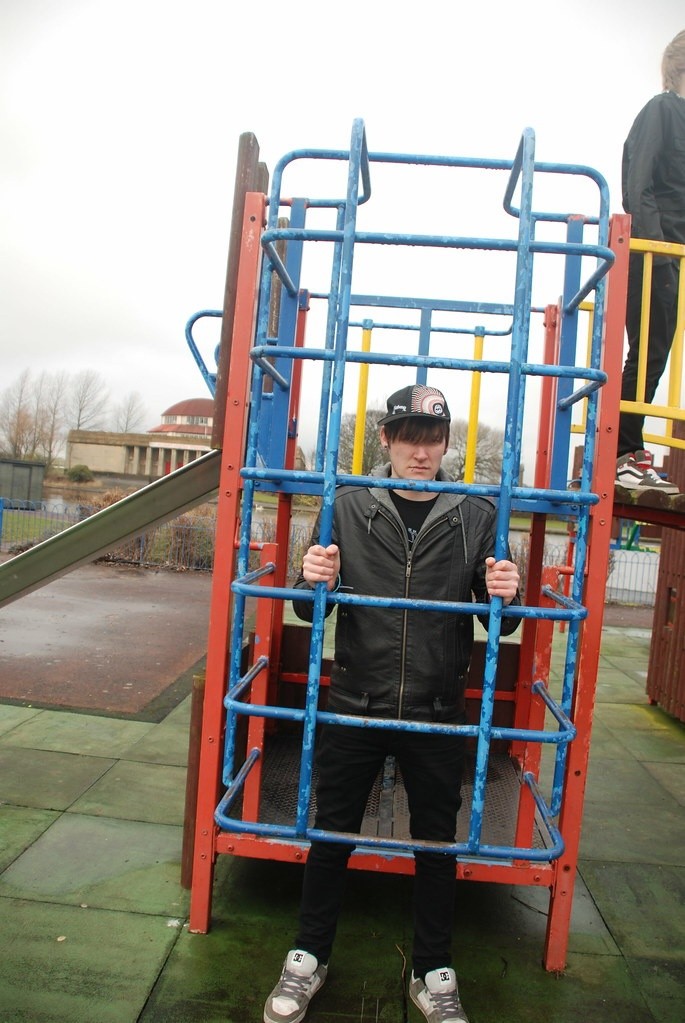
[
  {"x1": 263, "y1": 385, "x2": 520, "y2": 1023},
  {"x1": 614, "y1": 29, "x2": 685, "y2": 493}
]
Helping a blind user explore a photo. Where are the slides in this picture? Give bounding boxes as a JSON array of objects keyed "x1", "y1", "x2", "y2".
[{"x1": 1, "y1": 450, "x2": 223, "y2": 606}]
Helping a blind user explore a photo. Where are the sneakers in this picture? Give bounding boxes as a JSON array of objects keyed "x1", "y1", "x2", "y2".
[
  {"x1": 614, "y1": 450, "x2": 679, "y2": 493},
  {"x1": 408, "y1": 968, "x2": 469, "y2": 1023},
  {"x1": 263, "y1": 949, "x2": 329, "y2": 1023}
]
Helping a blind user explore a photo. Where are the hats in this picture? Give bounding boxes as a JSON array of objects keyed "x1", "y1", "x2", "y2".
[{"x1": 377, "y1": 385, "x2": 451, "y2": 425}]
